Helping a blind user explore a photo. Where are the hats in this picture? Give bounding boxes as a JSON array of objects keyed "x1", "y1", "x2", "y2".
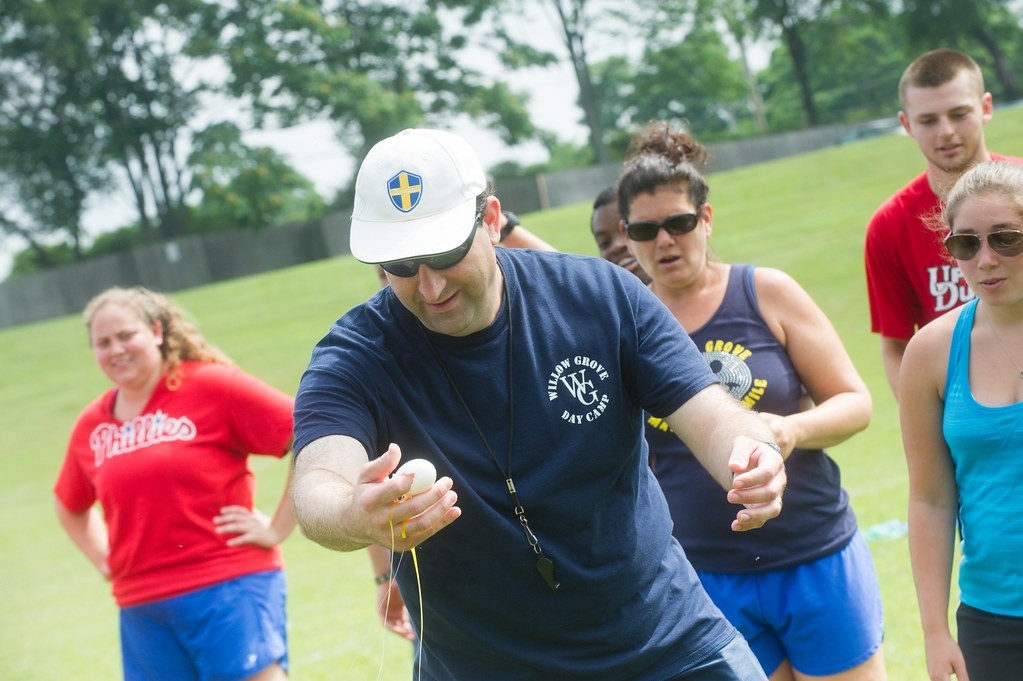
[{"x1": 349, "y1": 128, "x2": 486, "y2": 265}]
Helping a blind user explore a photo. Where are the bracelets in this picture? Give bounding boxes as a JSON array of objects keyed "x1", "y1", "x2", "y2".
[
  {"x1": 374, "y1": 570, "x2": 394, "y2": 585},
  {"x1": 499, "y1": 212, "x2": 520, "y2": 242}
]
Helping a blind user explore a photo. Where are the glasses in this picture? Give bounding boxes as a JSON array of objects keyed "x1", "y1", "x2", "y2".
[
  {"x1": 943, "y1": 231, "x2": 1023, "y2": 261},
  {"x1": 625, "y1": 205, "x2": 701, "y2": 243},
  {"x1": 378, "y1": 213, "x2": 482, "y2": 278}
]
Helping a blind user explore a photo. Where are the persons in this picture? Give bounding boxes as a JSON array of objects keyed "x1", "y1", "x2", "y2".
[
  {"x1": 897, "y1": 161, "x2": 1023, "y2": 680},
  {"x1": 291, "y1": 127, "x2": 788, "y2": 680},
  {"x1": 52, "y1": 286, "x2": 297, "y2": 680},
  {"x1": 588, "y1": 122, "x2": 885, "y2": 679},
  {"x1": 864, "y1": 47, "x2": 1022, "y2": 545}
]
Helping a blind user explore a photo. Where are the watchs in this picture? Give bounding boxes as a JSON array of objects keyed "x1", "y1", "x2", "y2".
[{"x1": 758, "y1": 439, "x2": 783, "y2": 457}]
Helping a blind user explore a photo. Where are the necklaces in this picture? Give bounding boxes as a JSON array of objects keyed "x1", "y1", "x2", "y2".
[{"x1": 978, "y1": 299, "x2": 1023, "y2": 379}]
